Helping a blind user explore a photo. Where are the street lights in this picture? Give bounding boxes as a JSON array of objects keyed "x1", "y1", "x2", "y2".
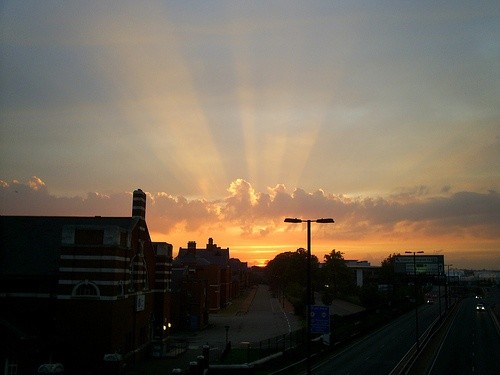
[
  {"x1": 407, "y1": 252, "x2": 425, "y2": 362},
  {"x1": 284, "y1": 216, "x2": 335, "y2": 374}
]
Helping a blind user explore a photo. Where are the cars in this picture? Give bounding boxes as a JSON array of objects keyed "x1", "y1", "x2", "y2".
[{"x1": 476, "y1": 304, "x2": 485, "y2": 310}]
